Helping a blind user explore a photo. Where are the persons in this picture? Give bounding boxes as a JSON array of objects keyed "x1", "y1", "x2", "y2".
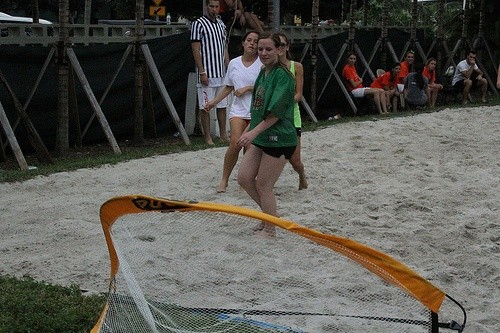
[
  {"x1": 236, "y1": 31, "x2": 298, "y2": 236},
  {"x1": 190, "y1": 0, "x2": 230, "y2": 147},
  {"x1": 219, "y1": 0, "x2": 264, "y2": 34},
  {"x1": 393, "y1": 50, "x2": 414, "y2": 115},
  {"x1": 403, "y1": 63, "x2": 429, "y2": 110},
  {"x1": 342, "y1": 53, "x2": 389, "y2": 116},
  {"x1": 276, "y1": 34, "x2": 308, "y2": 191},
  {"x1": 205, "y1": 30, "x2": 266, "y2": 193},
  {"x1": 422, "y1": 57, "x2": 442, "y2": 111},
  {"x1": 371, "y1": 62, "x2": 402, "y2": 112},
  {"x1": 452, "y1": 51, "x2": 488, "y2": 104}
]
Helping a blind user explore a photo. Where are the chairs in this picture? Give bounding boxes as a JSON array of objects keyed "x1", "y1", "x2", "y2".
[
  {"x1": 337, "y1": 81, "x2": 371, "y2": 118},
  {"x1": 435, "y1": 67, "x2": 472, "y2": 106}
]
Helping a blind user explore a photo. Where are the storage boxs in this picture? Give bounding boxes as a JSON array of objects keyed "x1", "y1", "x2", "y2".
[{"x1": 196, "y1": 88, "x2": 234, "y2": 136}]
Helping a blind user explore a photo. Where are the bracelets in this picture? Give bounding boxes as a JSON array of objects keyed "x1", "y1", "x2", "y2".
[{"x1": 200, "y1": 72, "x2": 205, "y2": 75}]
